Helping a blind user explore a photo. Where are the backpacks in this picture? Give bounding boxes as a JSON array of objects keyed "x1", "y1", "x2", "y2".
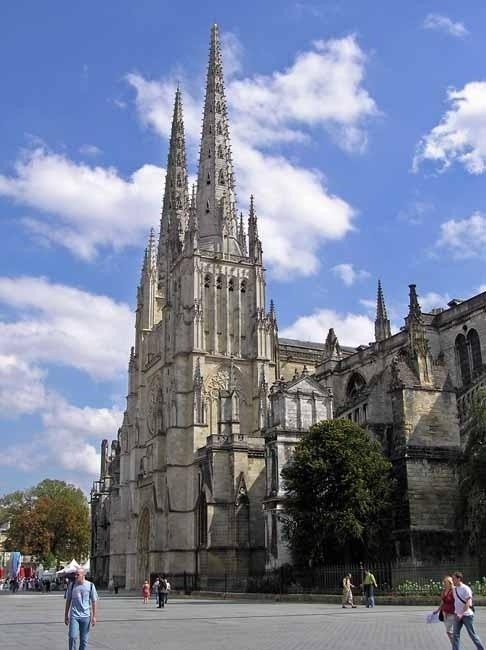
[{"x1": 160, "y1": 580, "x2": 167, "y2": 593}]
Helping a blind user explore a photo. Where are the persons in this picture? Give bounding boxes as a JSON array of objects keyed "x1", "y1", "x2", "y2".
[
  {"x1": 360, "y1": 569, "x2": 378, "y2": 608},
  {"x1": 113, "y1": 576, "x2": 118, "y2": 594},
  {"x1": 0, "y1": 574, "x2": 69, "y2": 594},
  {"x1": 451, "y1": 571, "x2": 484, "y2": 650},
  {"x1": 431, "y1": 576, "x2": 454, "y2": 642},
  {"x1": 143, "y1": 576, "x2": 170, "y2": 608},
  {"x1": 342, "y1": 572, "x2": 358, "y2": 609},
  {"x1": 65, "y1": 567, "x2": 97, "y2": 650}
]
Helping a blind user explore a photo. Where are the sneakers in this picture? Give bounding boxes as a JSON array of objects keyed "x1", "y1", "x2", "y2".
[
  {"x1": 366, "y1": 605, "x2": 374, "y2": 608},
  {"x1": 157, "y1": 605, "x2": 164, "y2": 608},
  {"x1": 342, "y1": 606, "x2": 357, "y2": 608}
]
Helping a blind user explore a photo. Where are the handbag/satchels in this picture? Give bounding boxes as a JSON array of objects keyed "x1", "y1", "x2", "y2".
[
  {"x1": 439, "y1": 611, "x2": 444, "y2": 621},
  {"x1": 470, "y1": 605, "x2": 475, "y2": 614}
]
